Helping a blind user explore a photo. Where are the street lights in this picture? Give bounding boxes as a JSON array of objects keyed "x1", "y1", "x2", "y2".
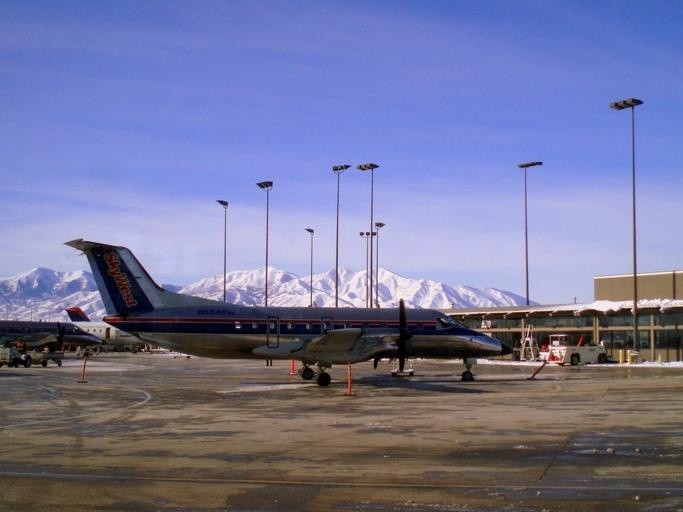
[
  {"x1": 255, "y1": 180, "x2": 272, "y2": 307},
  {"x1": 333, "y1": 165, "x2": 350, "y2": 307},
  {"x1": 216, "y1": 200, "x2": 227, "y2": 302},
  {"x1": 610, "y1": 98, "x2": 644, "y2": 350},
  {"x1": 518, "y1": 163, "x2": 542, "y2": 307},
  {"x1": 356, "y1": 163, "x2": 384, "y2": 309},
  {"x1": 305, "y1": 228, "x2": 314, "y2": 307}
]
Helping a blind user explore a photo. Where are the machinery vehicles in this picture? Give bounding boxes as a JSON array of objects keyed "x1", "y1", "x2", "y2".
[
  {"x1": 0, "y1": 344, "x2": 63, "y2": 367},
  {"x1": 547, "y1": 341, "x2": 609, "y2": 366}
]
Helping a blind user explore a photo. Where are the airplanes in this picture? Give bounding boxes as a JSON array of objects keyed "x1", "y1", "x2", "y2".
[
  {"x1": 0, "y1": 307, "x2": 145, "y2": 357},
  {"x1": 65, "y1": 239, "x2": 512, "y2": 386}
]
